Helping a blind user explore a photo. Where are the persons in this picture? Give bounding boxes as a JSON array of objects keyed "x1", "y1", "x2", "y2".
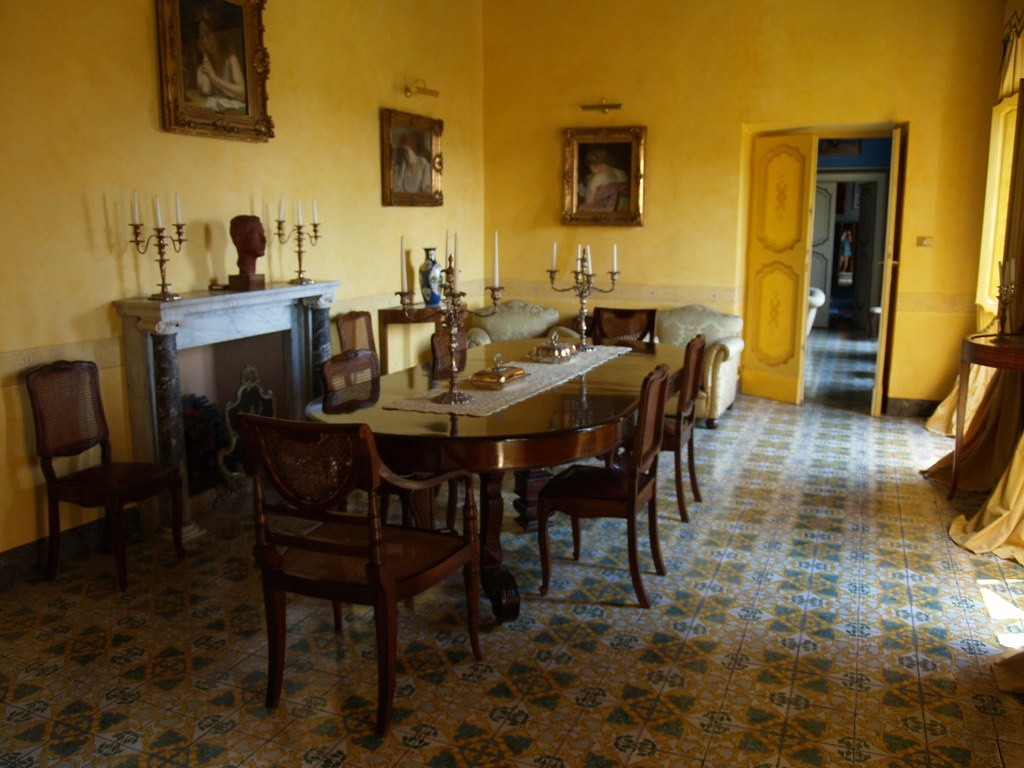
[
  {"x1": 228, "y1": 214, "x2": 266, "y2": 274},
  {"x1": 839, "y1": 229, "x2": 852, "y2": 273}
]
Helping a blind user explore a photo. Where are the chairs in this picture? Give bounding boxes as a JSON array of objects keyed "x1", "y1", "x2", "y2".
[
  {"x1": 331, "y1": 312, "x2": 380, "y2": 388},
  {"x1": 605, "y1": 333, "x2": 707, "y2": 522},
  {"x1": 321, "y1": 348, "x2": 379, "y2": 392},
  {"x1": 24, "y1": 359, "x2": 186, "y2": 591},
  {"x1": 536, "y1": 362, "x2": 670, "y2": 609},
  {"x1": 592, "y1": 307, "x2": 657, "y2": 345},
  {"x1": 380, "y1": 306, "x2": 442, "y2": 376},
  {"x1": 237, "y1": 411, "x2": 484, "y2": 740}
]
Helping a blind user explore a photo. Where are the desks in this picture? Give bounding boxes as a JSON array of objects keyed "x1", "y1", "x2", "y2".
[
  {"x1": 946, "y1": 331, "x2": 1024, "y2": 501},
  {"x1": 302, "y1": 338, "x2": 687, "y2": 625}
]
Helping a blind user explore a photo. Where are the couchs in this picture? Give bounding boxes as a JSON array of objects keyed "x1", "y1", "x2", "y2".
[{"x1": 467, "y1": 299, "x2": 744, "y2": 428}]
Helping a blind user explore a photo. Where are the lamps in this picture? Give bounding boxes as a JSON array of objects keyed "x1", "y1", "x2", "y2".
[
  {"x1": 546, "y1": 240, "x2": 620, "y2": 351},
  {"x1": 273, "y1": 191, "x2": 323, "y2": 286},
  {"x1": 990, "y1": 253, "x2": 1019, "y2": 343},
  {"x1": 130, "y1": 188, "x2": 188, "y2": 301},
  {"x1": 395, "y1": 229, "x2": 506, "y2": 405}
]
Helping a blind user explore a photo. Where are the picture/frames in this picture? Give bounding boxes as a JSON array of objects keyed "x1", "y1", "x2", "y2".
[
  {"x1": 157, "y1": 0, "x2": 275, "y2": 143},
  {"x1": 382, "y1": 108, "x2": 444, "y2": 206},
  {"x1": 561, "y1": 125, "x2": 647, "y2": 226}
]
